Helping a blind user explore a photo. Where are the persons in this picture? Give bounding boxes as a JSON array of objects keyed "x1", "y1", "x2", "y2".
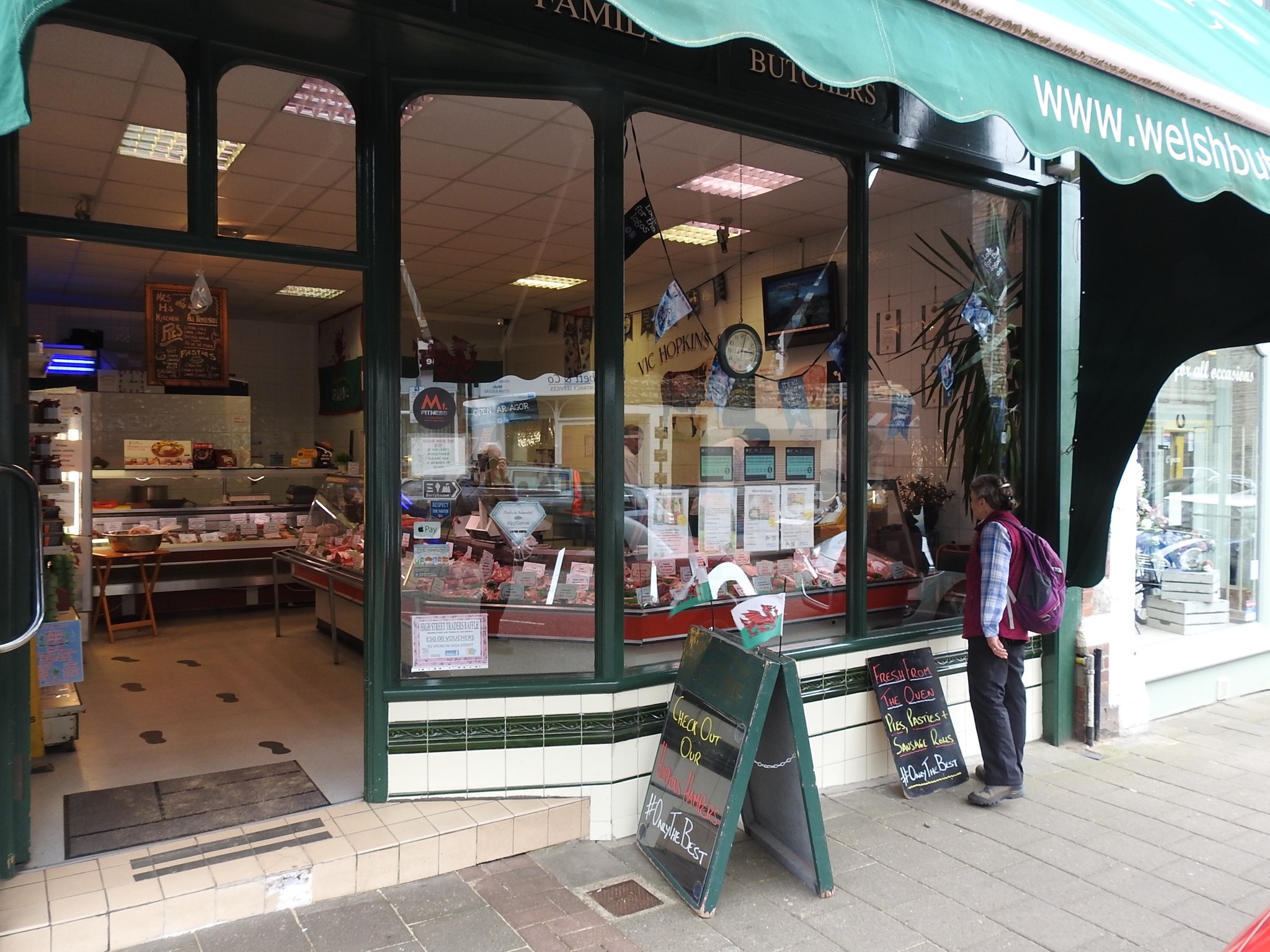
[
  {"x1": 623, "y1": 424, "x2": 644, "y2": 486},
  {"x1": 962, "y1": 474, "x2": 1065, "y2": 806},
  {"x1": 459, "y1": 444, "x2": 518, "y2": 655},
  {"x1": 689, "y1": 421, "x2": 770, "y2": 538}
]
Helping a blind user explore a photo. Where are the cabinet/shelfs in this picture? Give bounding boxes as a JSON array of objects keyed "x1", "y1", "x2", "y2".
[{"x1": 29, "y1": 422, "x2": 87, "y2": 754}]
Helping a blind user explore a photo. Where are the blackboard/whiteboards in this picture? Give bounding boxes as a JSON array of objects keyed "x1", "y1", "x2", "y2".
[
  {"x1": 145, "y1": 283, "x2": 229, "y2": 389},
  {"x1": 865, "y1": 647, "x2": 969, "y2": 800}
]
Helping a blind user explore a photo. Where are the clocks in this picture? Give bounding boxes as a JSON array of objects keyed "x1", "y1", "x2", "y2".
[
  {"x1": 119, "y1": 370, "x2": 148, "y2": 394},
  {"x1": 717, "y1": 323, "x2": 763, "y2": 379}
]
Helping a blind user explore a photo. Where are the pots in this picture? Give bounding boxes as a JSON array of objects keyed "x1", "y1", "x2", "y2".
[
  {"x1": 130, "y1": 485, "x2": 168, "y2": 501},
  {"x1": 145, "y1": 498, "x2": 197, "y2": 508}
]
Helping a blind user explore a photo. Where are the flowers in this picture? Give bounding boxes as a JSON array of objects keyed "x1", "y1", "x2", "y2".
[
  {"x1": 1165, "y1": 529, "x2": 1218, "y2": 573},
  {"x1": 1136, "y1": 462, "x2": 1169, "y2": 575}
]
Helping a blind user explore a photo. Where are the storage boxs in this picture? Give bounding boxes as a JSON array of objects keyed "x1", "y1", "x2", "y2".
[
  {"x1": 1144, "y1": 595, "x2": 1232, "y2": 636},
  {"x1": 1160, "y1": 568, "x2": 1223, "y2": 603},
  {"x1": 876, "y1": 522, "x2": 939, "y2": 571}
]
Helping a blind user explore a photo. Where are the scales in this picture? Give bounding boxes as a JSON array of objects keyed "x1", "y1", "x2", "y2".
[
  {"x1": 222, "y1": 476, "x2": 271, "y2": 506},
  {"x1": 464, "y1": 453, "x2": 552, "y2": 544}
]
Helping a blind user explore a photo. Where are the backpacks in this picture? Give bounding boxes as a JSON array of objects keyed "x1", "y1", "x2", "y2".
[{"x1": 993, "y1": 517, "x2": 1065, "y2": 635}]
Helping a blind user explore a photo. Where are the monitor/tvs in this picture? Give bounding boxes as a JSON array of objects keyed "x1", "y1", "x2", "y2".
[{"x1": 761, "y1": 260, "x2": 842, "y2": 352}]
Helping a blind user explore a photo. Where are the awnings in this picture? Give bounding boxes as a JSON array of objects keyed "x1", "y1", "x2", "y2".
[{"x1": 0, "y1": 0, "x2": 1270, "y2": 215}]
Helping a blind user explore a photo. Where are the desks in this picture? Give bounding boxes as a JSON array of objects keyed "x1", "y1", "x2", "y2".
[{"x1": 91, "y1": 546, "x2": 171, "y2": 644}]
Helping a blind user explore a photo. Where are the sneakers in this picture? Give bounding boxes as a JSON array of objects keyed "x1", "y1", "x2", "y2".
[
  {"x1": 976, "y1": 765, "x2": 988, "y2": 781},
  {"x1": 967, "y1": 785, "x2": 1024, "y2": 806}
]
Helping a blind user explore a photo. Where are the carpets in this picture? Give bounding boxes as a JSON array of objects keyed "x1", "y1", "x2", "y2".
[{"x1": 62, "y1": 759, "x2": 331, "y2": 860}]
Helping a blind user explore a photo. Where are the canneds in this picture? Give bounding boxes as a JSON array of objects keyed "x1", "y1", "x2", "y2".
[
  {"x1": 27, "y1": 334, "x2": 43, "y2": 354},
  {"x1": 25, "y1": 398, "x2": 63, "y2": 485}
]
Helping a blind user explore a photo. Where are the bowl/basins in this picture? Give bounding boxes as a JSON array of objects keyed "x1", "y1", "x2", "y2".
[{"x1": 101, "y1": 530, "x2": 168, "y2": 552}]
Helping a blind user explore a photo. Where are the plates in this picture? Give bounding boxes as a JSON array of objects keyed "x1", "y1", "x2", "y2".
[
  {"x1": 92, "y1": 500, "x2": 119, "y2": 508},
  {"x1": 151, "y1": 442, "x2": 184, "y2": 457}
]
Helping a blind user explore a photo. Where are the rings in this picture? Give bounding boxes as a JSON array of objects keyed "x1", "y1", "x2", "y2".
[{"x1": 993, "y1": 648, "x2": 996, "y2": 650}]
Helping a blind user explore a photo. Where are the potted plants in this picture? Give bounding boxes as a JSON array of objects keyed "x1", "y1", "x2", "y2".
[
  {"x1": 897, "y1": 474, "x2": 958, "y2": 533},
  {"x1": 334, "y1": 451, "x2": 354, "y2": 468}
]
